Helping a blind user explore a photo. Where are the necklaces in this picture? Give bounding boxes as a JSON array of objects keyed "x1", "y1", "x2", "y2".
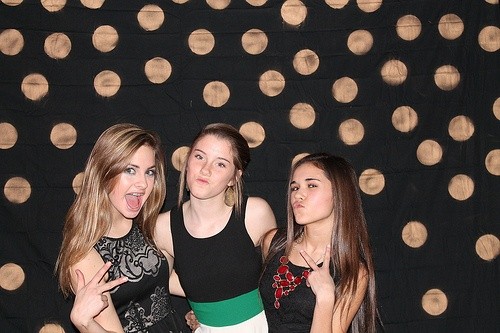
[{"x1": 271, "y1": 230, "x2": 325, "y2": 310}]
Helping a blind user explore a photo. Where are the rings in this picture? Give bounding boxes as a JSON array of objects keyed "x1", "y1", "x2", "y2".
[{"x1": 190, "y1": 325, "x2": 193, "y2": 330}]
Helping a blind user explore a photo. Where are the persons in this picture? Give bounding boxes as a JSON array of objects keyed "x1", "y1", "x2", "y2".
[
  {"x1": 151, "y1": 122, "x2": 279, "y2": 333},
  {"x1": 53, "y1": 123, "x2": 183, "y2": 333},
  {"x1": 183, "y1": 152, "x2": 385, "y2": 333}
]
